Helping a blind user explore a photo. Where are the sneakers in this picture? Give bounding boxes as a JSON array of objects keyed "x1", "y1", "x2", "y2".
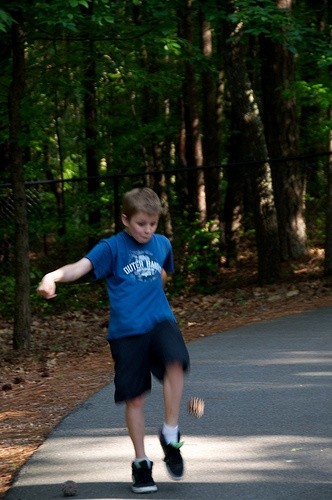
[
  {"x1": 159, "y1": 430, "x2": 184, "y2": 477},
  {"x1": 131, "y1": 460, "x2": 158, "y2": 493}
]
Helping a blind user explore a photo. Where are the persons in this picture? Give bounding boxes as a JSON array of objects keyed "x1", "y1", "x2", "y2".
[{"x1": 37, "y1": 188, "x2": 190, "y2": 492}]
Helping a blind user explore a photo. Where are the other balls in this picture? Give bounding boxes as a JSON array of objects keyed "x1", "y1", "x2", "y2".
[{"x1": 62, "y1": 480, "x2": 78, "y2": 497}]
